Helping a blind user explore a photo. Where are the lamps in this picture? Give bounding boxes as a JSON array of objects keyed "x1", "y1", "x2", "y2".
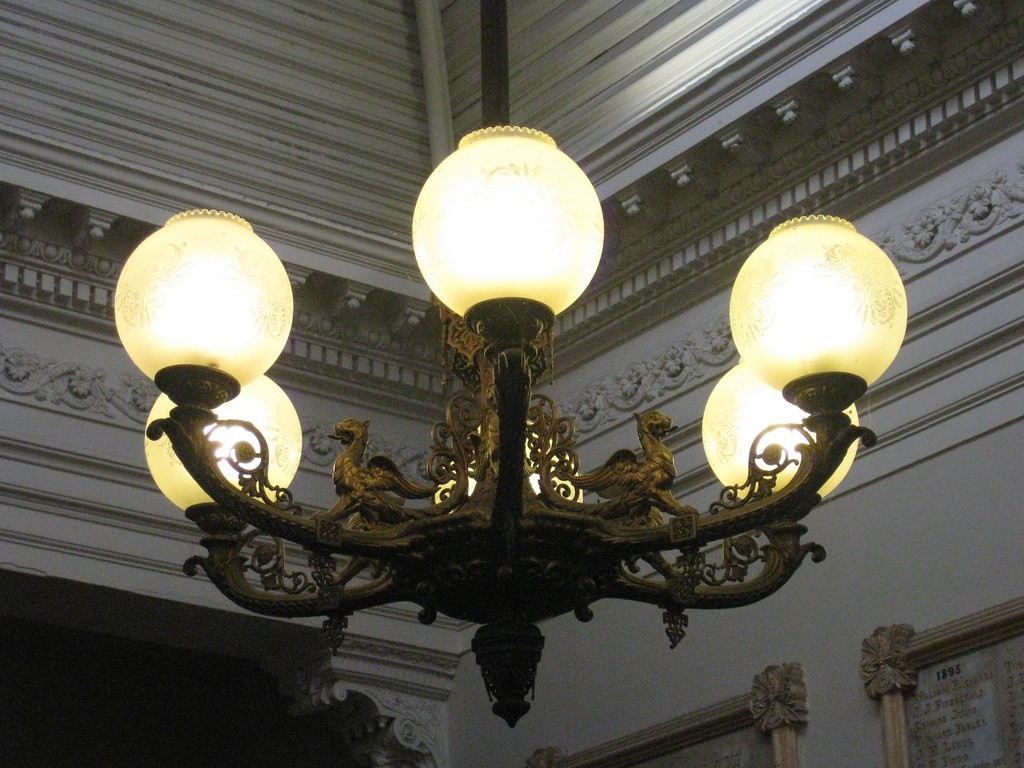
[{"x1": 114, "y1": 0, "x2": 908, "y2": 730}]
